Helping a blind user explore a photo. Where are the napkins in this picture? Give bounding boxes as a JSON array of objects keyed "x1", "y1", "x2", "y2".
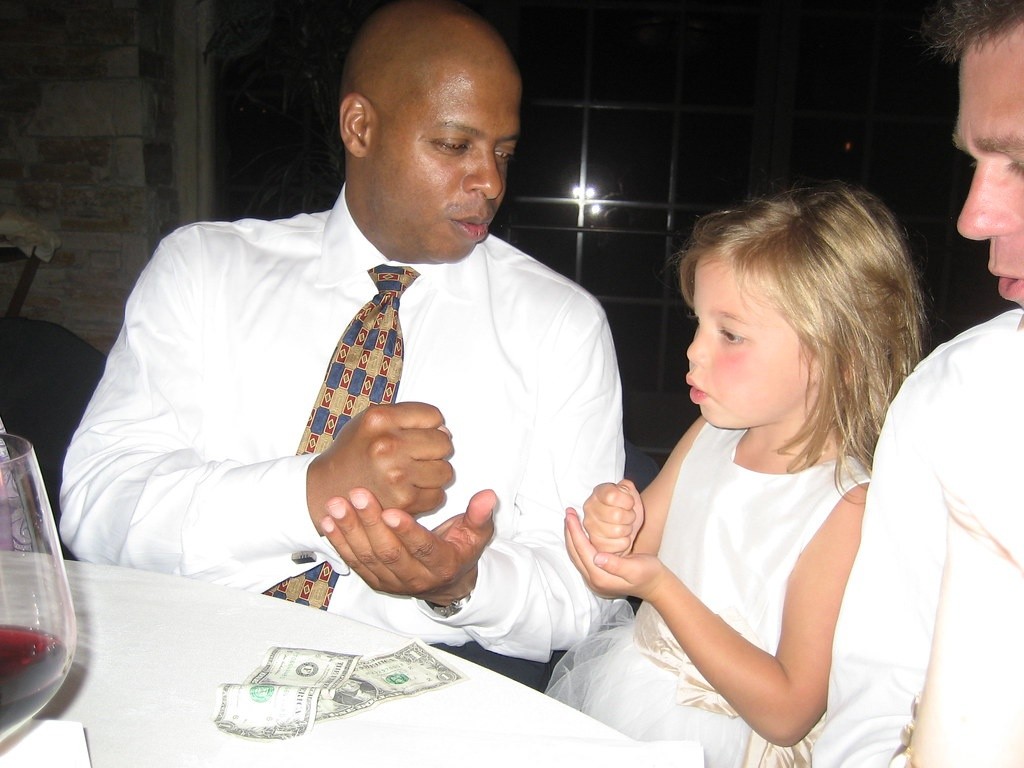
[
  {"x1": 0, "y1": 721, "x2": 93, "y2": 768},
  {"x1": 529, "y1": 740, "x2": 706, "y2": 768}
]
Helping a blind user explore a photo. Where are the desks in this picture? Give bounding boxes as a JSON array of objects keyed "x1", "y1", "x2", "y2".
[{"x1": 0, "y1": 548, "x2": 677, "y2": 768}]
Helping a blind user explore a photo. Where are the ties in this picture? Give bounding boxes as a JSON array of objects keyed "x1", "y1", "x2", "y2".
[{"x1": 262, "y1": 264, "x2": 422, "y2": 611}]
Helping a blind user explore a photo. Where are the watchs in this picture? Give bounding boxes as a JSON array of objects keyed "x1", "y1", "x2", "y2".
[{"x1": 424, "y1": 592, "x2": 471, "y2": 619}]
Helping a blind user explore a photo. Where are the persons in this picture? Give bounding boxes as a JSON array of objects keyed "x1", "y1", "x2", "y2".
[
  {"x1": 564, "y1": 184, "x2": 925, "y2": 768},
  {"x1": 59, "y1": 1, "x2": 627, "y2": 697},
  {"x1": 814, "y1": 0, "x2": 1024, "y2": 768}
]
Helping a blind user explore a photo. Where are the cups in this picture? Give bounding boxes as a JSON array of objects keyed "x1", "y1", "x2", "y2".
[{"x1": 0, "y1": 431, "x2": 75, "y2": 744}]
